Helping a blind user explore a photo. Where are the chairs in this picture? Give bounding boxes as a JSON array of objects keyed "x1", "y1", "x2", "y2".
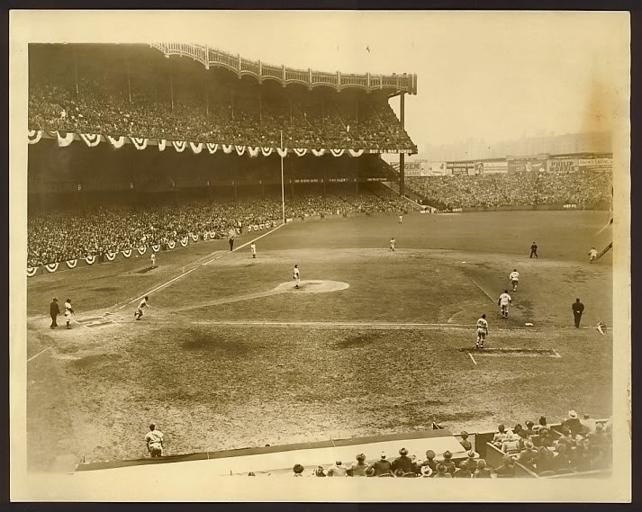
[{"x1": 362, "y1": 415, "x2": 613, "y2": 479}]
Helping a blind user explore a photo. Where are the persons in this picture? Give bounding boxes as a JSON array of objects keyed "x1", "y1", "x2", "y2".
[
  {"x1": 292, "y1": 264, "x2": 302, "y2": 290},
  {"x1": 134, "y1": 296, "x2": 151, "y2": 320},
  {"x1": 28, "y1": 62, "x2": 615, "y2": 268},
  {"x1": 48, "y1": 298, "x2": 61, "y2": 329},
  {"x1": 474, "y1": 315, "x2": 490, "y2": 349},
  {"x1": 248, "y1": 410, "x2": 613, "y2": 482},
  {"x1": 509, "y1": 269, "x2": 521, "y2": 290},
  {"x1": 498, "y1": 289, "x2": 512, "y2": 320},
  {"x1": 570, "y1": 298, "x2": 585, "y2": 327},
  {"x1": 146, "y1": 423, "x2": 164, "y2": 459},
  {"x1": 63, "y1": 298, "x2": 76, "y2": 329}
]
